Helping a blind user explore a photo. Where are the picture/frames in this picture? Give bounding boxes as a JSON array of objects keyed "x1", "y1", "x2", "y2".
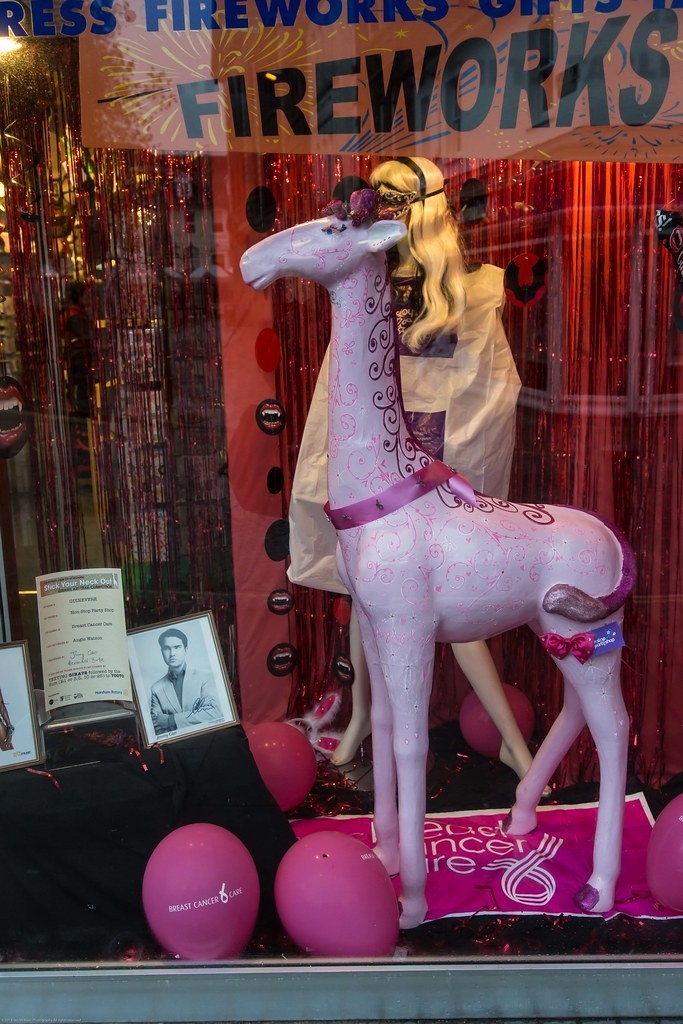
[
  {"x1": 125, "y1": 609, "x2": 240, "y2": 749},
  {"x1": 0, "y1": 638, "x2": 44, "y2": 772}
]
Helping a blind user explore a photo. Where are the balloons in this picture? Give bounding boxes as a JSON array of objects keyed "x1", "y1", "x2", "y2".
[
  {"x1": 246, "y1": 723, "x2": 315, "y2": 810},
  {"x1": 461, "y1": 684, "x2": 533, "y2": 760},
  {"x1": 274, "y1": 832, "x2": 399, "y2": 957},
  {"x1": 143, "y1": 823, "x2": 259, "y2": 961},
  {"x1": 646, "y1": 792, "x2": 683, "y2": 912}
]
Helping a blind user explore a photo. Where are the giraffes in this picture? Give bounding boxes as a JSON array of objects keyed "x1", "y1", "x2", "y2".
[{"x1": 240, "y1": 188, "x2": 640, "y2": 931}]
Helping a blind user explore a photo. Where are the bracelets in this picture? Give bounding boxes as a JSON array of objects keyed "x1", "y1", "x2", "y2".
[{"x1": 7, "y1": 726, "x2": 15, "y2": 730}]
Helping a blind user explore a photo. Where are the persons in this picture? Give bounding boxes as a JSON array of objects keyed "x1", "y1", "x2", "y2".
[
  {"x1": 150, "y1": 628, "x2": 223, "y2": 736},
  {"x1": 284, "y1": 160, "x2": 555, "y2": 797},
  {"x1": 0, "y1": 688, "x2": 15, "y2": 750}
]
